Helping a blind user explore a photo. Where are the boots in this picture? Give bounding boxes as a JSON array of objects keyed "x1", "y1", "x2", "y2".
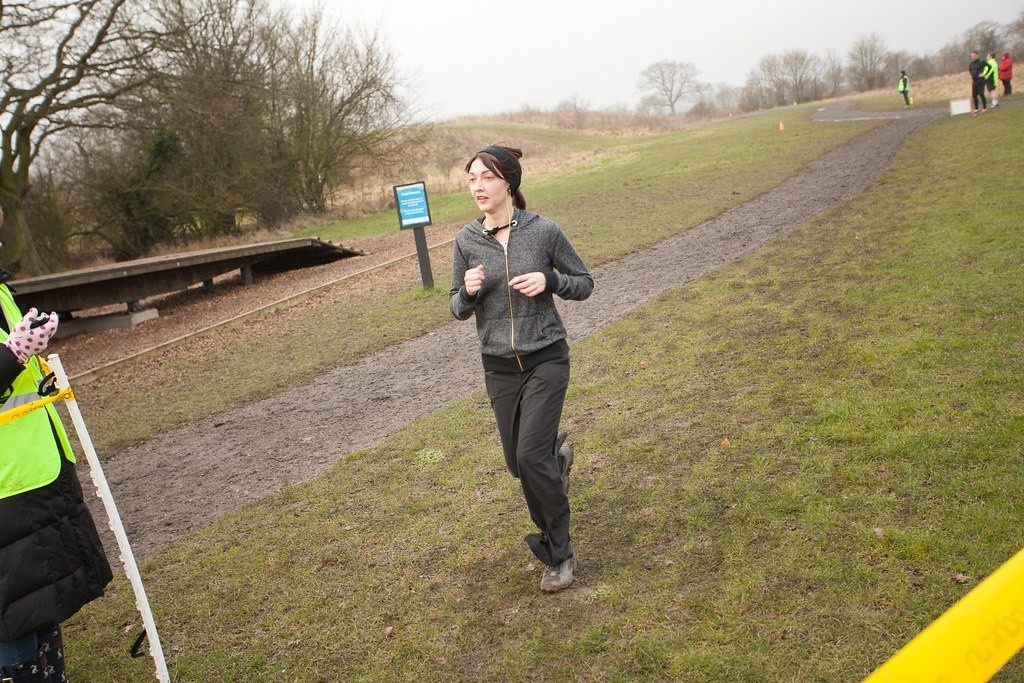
[
  {"x1": 37, "y1": 624, "x2": 69, "y2": 683},
  {"x1": 0, "y1": 644, "x2": 52, "y2": 683}
]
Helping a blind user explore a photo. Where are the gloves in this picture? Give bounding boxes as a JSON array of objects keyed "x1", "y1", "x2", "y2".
[{"x1": 6, "y1": 308, "x2": 58, "y2": 363}]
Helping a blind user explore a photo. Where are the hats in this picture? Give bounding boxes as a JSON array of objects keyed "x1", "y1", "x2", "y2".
[{"x1": 1003, "y1": 52, "x2": 1010, "y2": 58}]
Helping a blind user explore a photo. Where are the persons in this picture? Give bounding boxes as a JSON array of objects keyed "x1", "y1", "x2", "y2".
[
  {"x1": 969, "y1": 51, "x2": 989, "y2": 117},
  {"x1": 0, "y1": 205, "x2": 115, "y2": 683},
  {"x1": 447, "y1": 139, "x2": 594, "y2": 593},
  {"x1": 983, "y1": 52, "x2": 999, "y2": 109},
  {"x1": 899, "y1": 71, "x2": 911, "y2": 109},
  {"x1": 999, "y1": 51, "x2": 1013, "y2": 96}
]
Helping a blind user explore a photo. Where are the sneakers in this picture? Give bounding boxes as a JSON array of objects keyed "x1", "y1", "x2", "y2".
[
  {"x1": 541, "y1": 547, "x2": 576, "y2": 593},
  {"x1": 557, "y1": 442, "x2": 574, "y2": 496}
]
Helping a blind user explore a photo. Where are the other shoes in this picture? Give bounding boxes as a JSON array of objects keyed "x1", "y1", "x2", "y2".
[
  {"x1": 982, "y1": 109, "x2": 987, "y2": 113},
  {"x1": 972, "y1": 110, "x2": 979, "y2": 118},
  {"x1": 991, "y1": 100, "x2": 998, "y2": 109}
]
